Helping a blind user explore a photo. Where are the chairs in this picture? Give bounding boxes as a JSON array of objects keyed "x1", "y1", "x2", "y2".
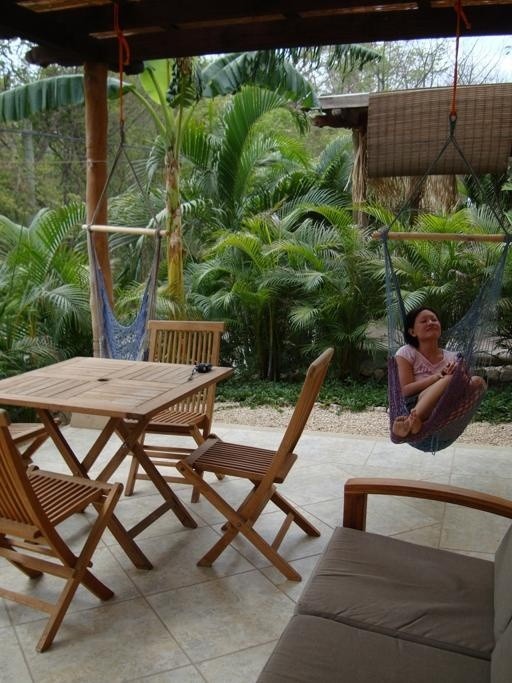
[
  {"x1": 178, "y1": 346, "x2": 337, "y2": 583},
  {"x1": 0, "y1": 407, "x2": 125, "y2": 653},
  {"x1": 125, "y1": 317, "x2": 228, "y2": 503}
]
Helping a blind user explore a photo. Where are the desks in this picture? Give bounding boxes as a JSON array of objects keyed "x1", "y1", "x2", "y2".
[{"x1": 0, "y1": 351, "x2": 235, "y2": 574}]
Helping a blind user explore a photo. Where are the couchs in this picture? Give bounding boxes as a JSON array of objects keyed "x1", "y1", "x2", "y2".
[{"x1": 262, "y1": 474, "x2": 512, "y2": 682}]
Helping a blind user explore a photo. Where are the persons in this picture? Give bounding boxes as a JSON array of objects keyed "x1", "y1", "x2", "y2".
[{"x1": 392, "y1": 306, "x2": 487, "y2": 452}]
